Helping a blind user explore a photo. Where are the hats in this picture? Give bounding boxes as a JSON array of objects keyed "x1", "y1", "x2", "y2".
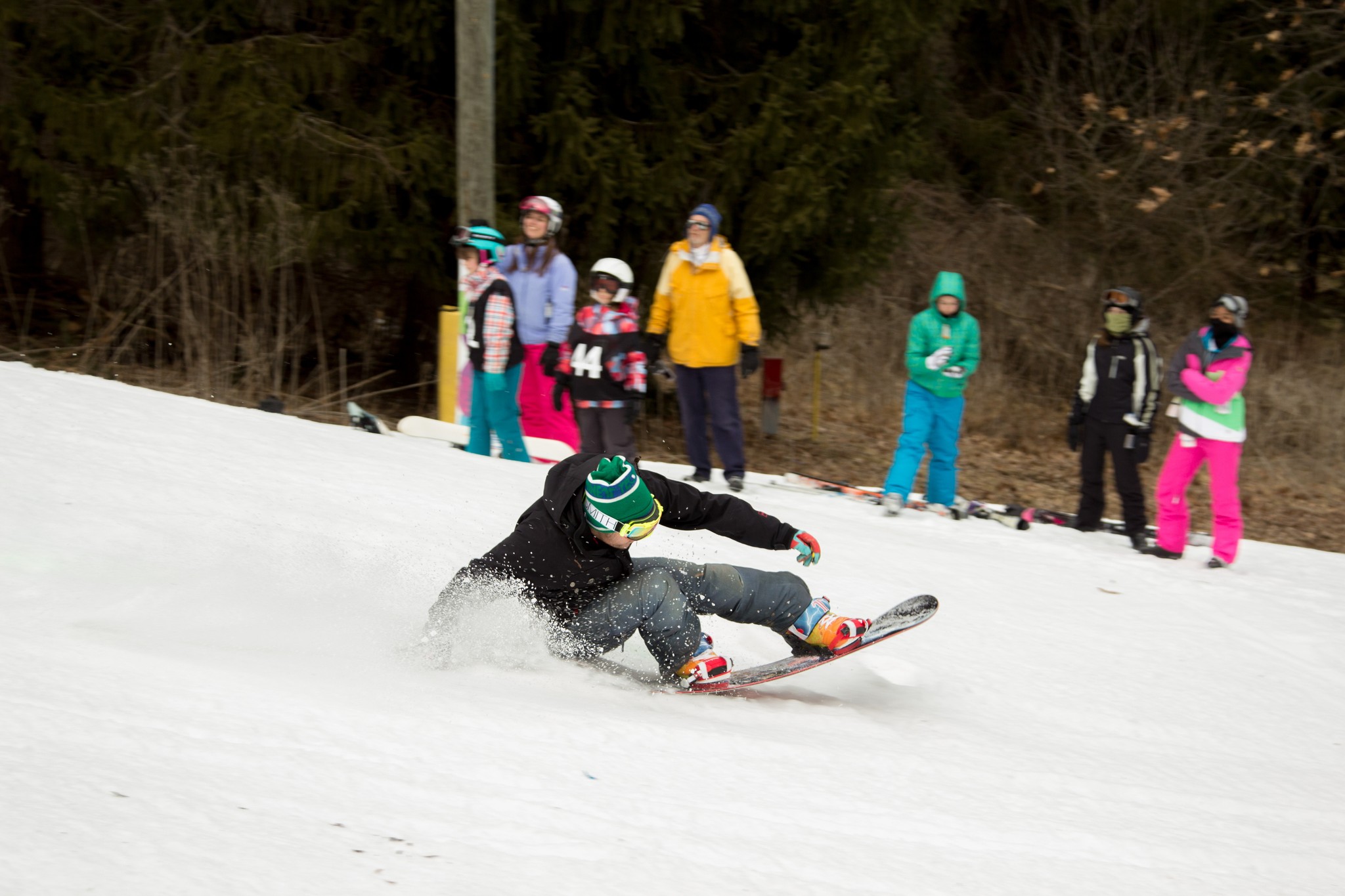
[
  {"x1": 689, "y1": 204, "x2": 722, "y2": 243},
  {"x1": 585, "y1": 455, "x2": 654, "y2": 533},
  {"x1": 1210, "y1": 292, "x2": 1250, "y2": 326}
]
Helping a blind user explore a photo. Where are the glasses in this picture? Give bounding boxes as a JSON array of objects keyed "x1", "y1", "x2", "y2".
[
  {"x1": 452, "y1": 227, "x2": 497, "y2": 244},
  {"x1": 584, "y1": 495, "x2": 663, "y2": 542},
  {"x1": 1101, "y1": 289, "x2": 1138, "y2": 308},
  {"x1": 1212, "y1": 295, "x2": 1240, "y2": 313},
  {"x1": 682, "y1": 219, "x2": 710, "y2": 231},
  {"x1": 590, "y1": 273, "x2": 634, "y2": 293}
]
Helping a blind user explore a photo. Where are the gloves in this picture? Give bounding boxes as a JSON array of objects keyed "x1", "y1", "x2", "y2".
[
  {"x1": 479, "y1": 338, "x2": 763, "y2": 420},
  {"x1": 791, "y1": 528, "x2": 820, "y2": 567},
  {"x1": 1124, "y1": 431, "x2": 1148, "y2": 465},
  {"x1": 927, "y1": 346, "x2": 966, "y2": 381},
  {"x1": 1066, "y1": 422, "x2": 1083, "y2": 451}
]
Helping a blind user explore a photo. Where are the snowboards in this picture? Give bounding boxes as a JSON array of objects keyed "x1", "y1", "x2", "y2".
[{"x1": 662, "y1": 595, "x2": 939, "y2": 698}]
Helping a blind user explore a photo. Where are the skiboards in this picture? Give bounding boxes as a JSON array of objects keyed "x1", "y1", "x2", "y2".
[
  {"x1": 346, "y1": 400, "x2": 579, "y2": 463},
  {"x1": 781, "y1": 471, "x2": 1220, "y2": 549}
]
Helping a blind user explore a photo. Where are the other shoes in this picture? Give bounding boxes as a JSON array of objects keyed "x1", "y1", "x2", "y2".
[
  {"x1": 673, "y1": 632, "x2": 733, "y2": 688},
  {"x1": 1140, "y1": 546, "x2": 1231, "y2": 568},
  {"x1": 684, "y1": 471, "x2": 745, "y2": 493},
  {"x1": 1070, "y1": 516, "x2": 1148, "y2": 548},
  {"x1": 880, "y1": 494, "x2": 957, "y2": 521},
  {"x1": 783, "y1": 596, "x2": 872, "y2": 660}
]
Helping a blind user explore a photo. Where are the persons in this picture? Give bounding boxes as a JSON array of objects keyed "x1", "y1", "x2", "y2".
[
  {"x1": 448, "y1": 225, "x2": 531, "y2": 463},
  {"x1": 881, "y1": 271, "x2": 981, "y2": 517},
  {"x1": 554, "y1": 257, "x2": 650, "y2": 474},
  {"x1": 644, "y1": 203, "x2": 762, "y2": 492},
  {"x1": 422, "y1": 453, "x2": 873, "y2": 685},
  {"x1": 1141, "y1": 294, "x2": 1253, "y2": 568},
  {"x1": 487, "y1": 195, "x2": 580, "y2": 465},
  {"x1": 1066, "y1": 284, "x2": 1160, "y2": 551}
]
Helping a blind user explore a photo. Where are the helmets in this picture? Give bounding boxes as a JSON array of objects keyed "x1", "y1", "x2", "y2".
[
  {"x1": 520, "y1": 196, "x2": 563, "y2": 238},
  {"x1": 451, "y1": 223, "x2": 505, "y2": 265},
  {"x1": 589, "y1": 259, "x2": 634, "y2": 303},
  {"x1": 1102, "y1": 286, "x2": 1146, "y2": 317}
]
